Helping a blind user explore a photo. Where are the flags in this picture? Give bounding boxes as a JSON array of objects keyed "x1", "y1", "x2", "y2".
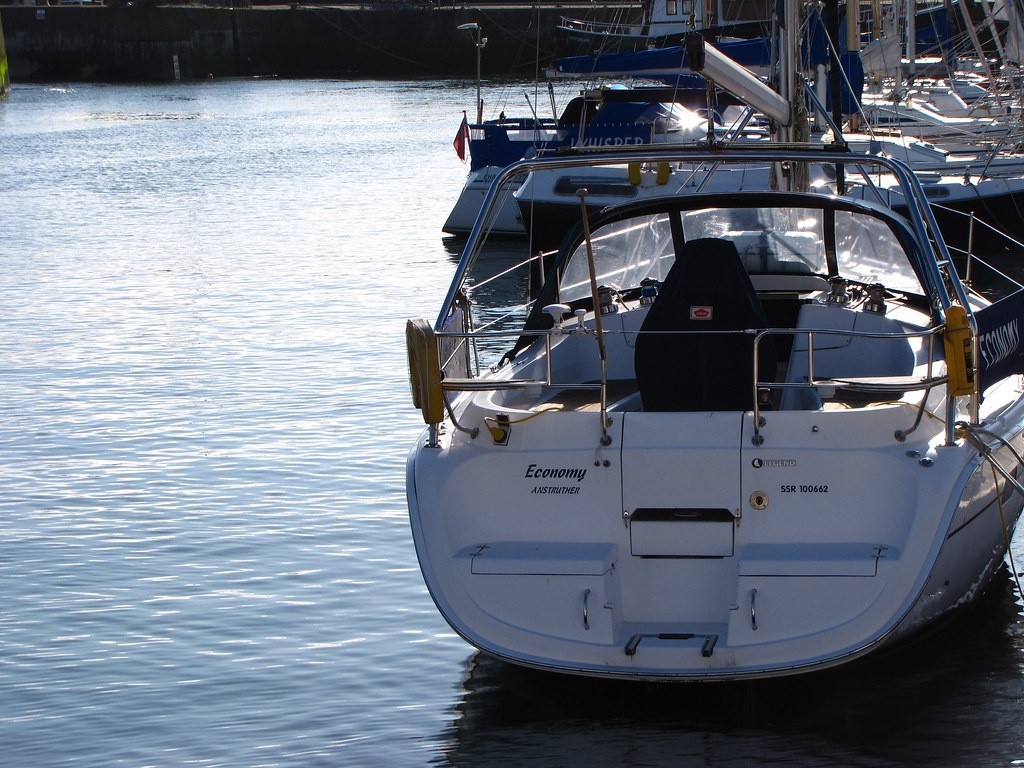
[{"x1": 452, "y1": 116, "x2": 471, "y2": 164}]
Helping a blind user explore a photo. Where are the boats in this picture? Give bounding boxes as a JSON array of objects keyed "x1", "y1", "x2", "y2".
[{"x1": 403, "y1": 141, "x2": 1024, "y2": 683}]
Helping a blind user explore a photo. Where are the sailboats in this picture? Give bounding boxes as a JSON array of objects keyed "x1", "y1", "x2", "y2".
[{"x1": 389, "y1": 0, "x2": 1024, "y2": 255}]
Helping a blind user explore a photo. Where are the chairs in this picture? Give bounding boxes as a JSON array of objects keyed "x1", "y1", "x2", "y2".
[{"x1": 634, "y1": 239, "x2": 779, "y2": 412}]
